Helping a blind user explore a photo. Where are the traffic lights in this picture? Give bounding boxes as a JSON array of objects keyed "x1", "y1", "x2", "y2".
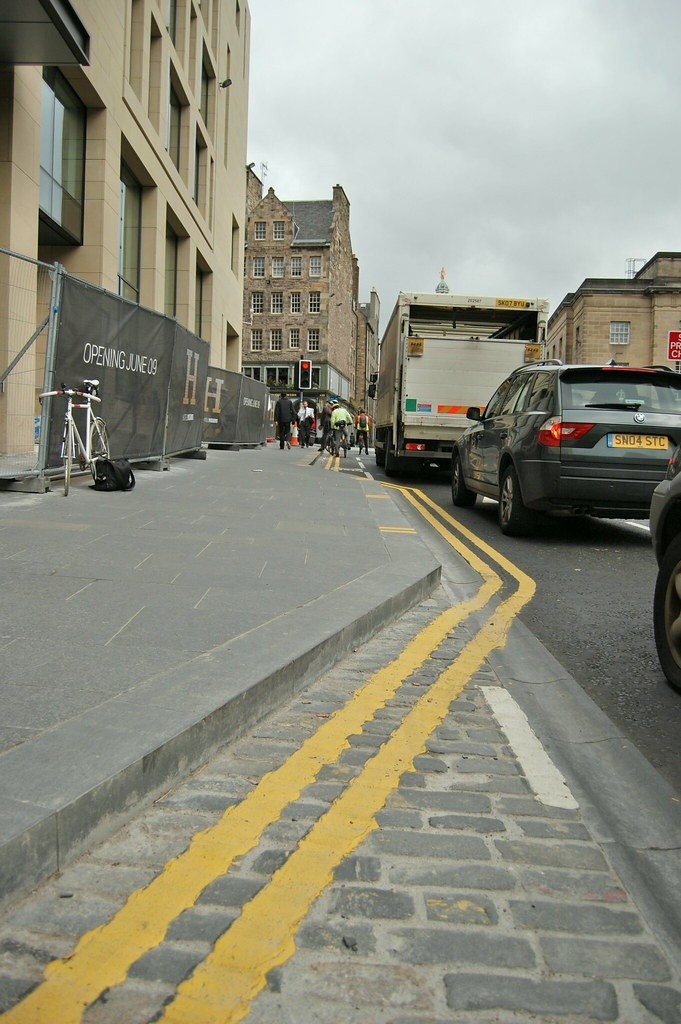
[{"x1": 298, "y1": 360, "x2": 312, "y2": 390}]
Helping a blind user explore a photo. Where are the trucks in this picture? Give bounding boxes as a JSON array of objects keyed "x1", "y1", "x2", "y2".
[{"x1": 367, "y1": 289, "x2": 551, "y2": 481}]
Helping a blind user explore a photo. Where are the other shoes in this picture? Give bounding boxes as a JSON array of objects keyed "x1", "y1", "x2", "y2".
[
  {"x1": 365, "y1": 450, "x2": 369, "y2": 455},
  {"x1": 287, "y1": 442, "x2": 291, "y2": 450}
]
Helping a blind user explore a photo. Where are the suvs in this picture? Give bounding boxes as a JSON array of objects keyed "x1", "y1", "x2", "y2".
[{"x1": 449, "y1": 358, "x2": 681, "y2": 539}]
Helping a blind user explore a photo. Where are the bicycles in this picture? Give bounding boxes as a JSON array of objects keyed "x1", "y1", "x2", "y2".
[
  {"x1": 354, "y1": 427, "x2": 368, "y2": 455},
  {"x1": 307, "y1": 423, "x2": 350, "y2": 458},
  {"x1": 38, "y1": 381, "x2": 112, "y2": 497}
]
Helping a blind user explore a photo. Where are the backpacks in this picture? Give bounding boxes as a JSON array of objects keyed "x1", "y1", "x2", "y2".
[{"x1": 360, "y1": 415, "x2": 366, "y2": 428}]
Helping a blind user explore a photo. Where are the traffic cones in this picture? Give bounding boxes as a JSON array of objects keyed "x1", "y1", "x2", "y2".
[{"x1": 290, "y1": 421, "x2": 301, "y2": 447}]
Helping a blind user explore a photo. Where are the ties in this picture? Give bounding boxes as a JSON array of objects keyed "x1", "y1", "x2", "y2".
[{"x1": 305, "y1": 409, "x2": 306, "y2": 416}]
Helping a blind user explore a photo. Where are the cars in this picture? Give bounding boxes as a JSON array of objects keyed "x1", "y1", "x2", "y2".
[{"x1": 650, "y1": 441, "x2": 681, "y2": 695}]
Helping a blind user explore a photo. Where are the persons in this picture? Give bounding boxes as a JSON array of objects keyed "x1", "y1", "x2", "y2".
[
  {"x1": 318, "y1": 400, "x2": 353, "y2": 457},
  {"x1": 354, "y1": 409, "x2": 371, "y2": 455},
  {"x1": 274, "y1": 391, "x2": 296, "y2": 450},
  {"x1": 297, "y1": 400, "x2": 315, "y2": 448}
]
